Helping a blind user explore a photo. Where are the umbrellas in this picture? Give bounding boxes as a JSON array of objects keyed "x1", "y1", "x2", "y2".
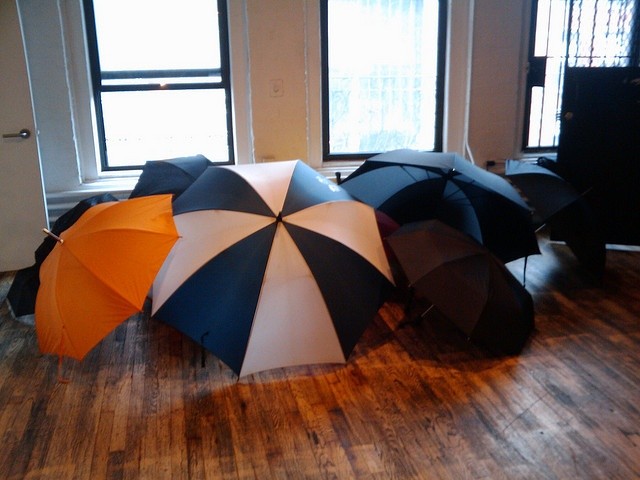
[
  {"x1": 7, "y1": 193, "x2": 119, "y2": 318},
  {"x1": 337, "y1": 149, "x2": 542, "y2": 265},
  {"x1": 384, "y1": 219, "x2": 533, "y2": 354},
  {"x1": 504, "y1": 159, "x2": 606, "y2": 274},
  {"x1": 537, "y1": 156, "x2": 583, "y2": 195},
  {"x1": 353, "y1": 195, "x2": 402, "y2": 253},
  {"x1": 34, "y1": 192, "x2": 183, "y2": 362},
  {"x1": 150, "y1": 158, "x2": 398, "y2": 382},
  {"x1": 128, "y1": 154, "x2": 215, "y2": 203}
]
